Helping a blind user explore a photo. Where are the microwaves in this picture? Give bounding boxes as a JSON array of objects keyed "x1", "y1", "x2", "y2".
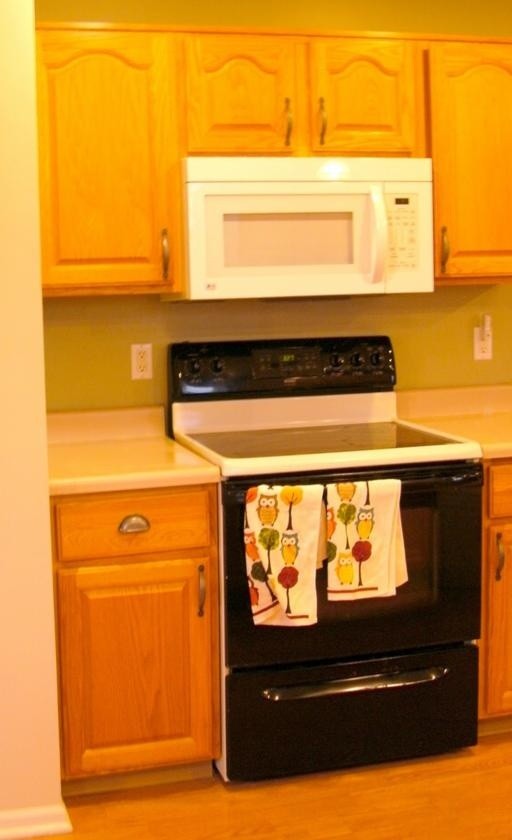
[{"x1": 159, "y1": 156, "x2": 437, "y2": 304}]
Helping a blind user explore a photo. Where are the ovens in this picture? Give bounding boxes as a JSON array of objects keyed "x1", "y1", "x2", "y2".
[{"x1": 213, "y1": 463, "x2": 482, "y2": 783}]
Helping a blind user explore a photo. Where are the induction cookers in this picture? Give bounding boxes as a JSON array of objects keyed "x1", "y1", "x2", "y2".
[{"x1": 177, "y1": 420, "x2": 482, "y2": 477}]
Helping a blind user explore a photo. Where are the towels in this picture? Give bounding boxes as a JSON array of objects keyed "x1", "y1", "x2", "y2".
[{"x1": 243, "y1": 481, "x2": 410, "y2": 633}]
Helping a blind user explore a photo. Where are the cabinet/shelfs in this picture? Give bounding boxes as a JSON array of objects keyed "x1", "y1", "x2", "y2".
[
  {"x1": 427, "y1": 41, "x2": 512, "y2": 279},
  {"x1": 35, "y1": 29, "x2": 182, "y2": 299},
  {"x1": 479, "y1": 464, "x2": 512, "y2": 719},
  {"x1": 52, "y1": 485, "x2": 221, "y2": 781},
  {"x1": 183, "y1": 35, "x2": 423, "y2": 159}
]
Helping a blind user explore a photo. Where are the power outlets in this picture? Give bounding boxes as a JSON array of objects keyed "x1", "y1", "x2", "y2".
[
  {"x1": 472, "y1": 327, "x2": 492, "y2": 360},
  {"x1": 131, "y1": 343, "x2": 153, "y2": 380}
]
